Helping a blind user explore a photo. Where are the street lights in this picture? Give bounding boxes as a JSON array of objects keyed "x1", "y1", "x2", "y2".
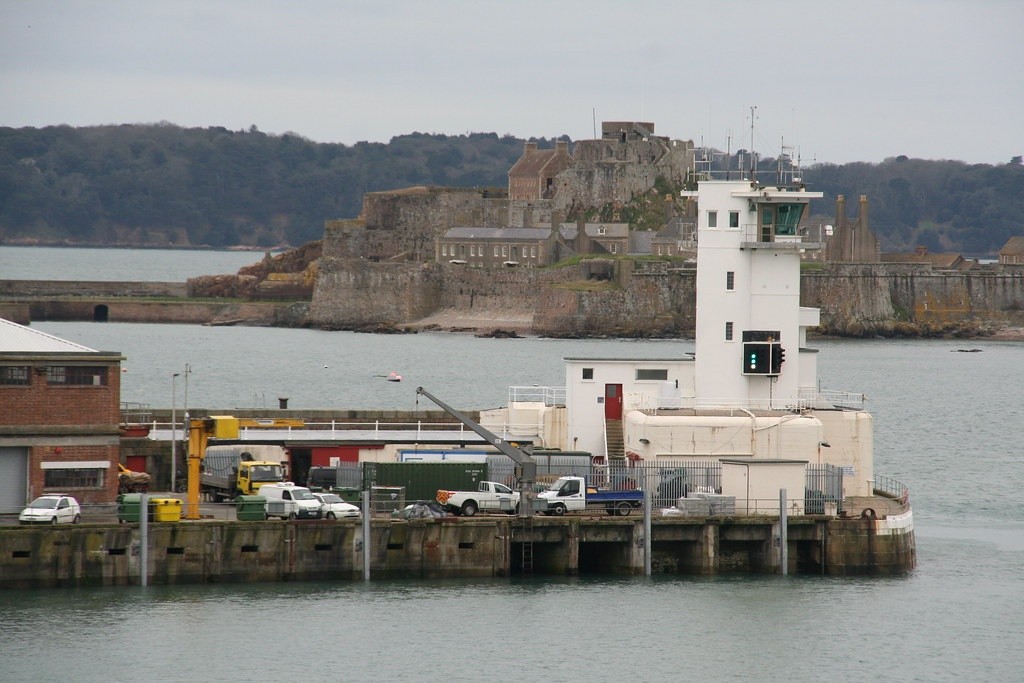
[{"x1": 171, "y1": 374, "x2": 180, "y2": 493}]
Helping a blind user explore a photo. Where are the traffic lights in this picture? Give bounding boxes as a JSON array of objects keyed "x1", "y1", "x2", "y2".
[
  {"x1": 772, "y1": 344, "x2": 786, "y2": 373},
  {"x1": 745, "y1": 344, "x2": 770, "y2": 373}
]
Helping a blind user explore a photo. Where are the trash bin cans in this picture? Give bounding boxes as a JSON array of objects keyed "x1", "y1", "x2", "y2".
[
  {"x1": 151, "y1": 499, "x2": 184, "y2": 523},
  {"x1": 115, "y1": 493, "x2": 171, "y2": 524},
  {"x1": 234, "y1": 495, "x2": 268, "y2": 521}
]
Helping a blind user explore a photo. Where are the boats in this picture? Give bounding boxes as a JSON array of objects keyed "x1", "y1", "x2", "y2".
[{"x1": 387, "y1": 372, "x2": 402, "y2": 381}]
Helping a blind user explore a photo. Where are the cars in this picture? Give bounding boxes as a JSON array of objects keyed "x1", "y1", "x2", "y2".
[
  {"x1": 19, "y1": 493, "x2": 81, "y2": 524},
  {"x1": 305, "y1": 492, "x2": 361, "y2": 519}
]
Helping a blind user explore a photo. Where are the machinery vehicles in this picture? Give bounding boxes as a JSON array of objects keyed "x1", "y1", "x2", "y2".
[{"x1": 416, "y1": 387, "x2": 538, "y2": 515}]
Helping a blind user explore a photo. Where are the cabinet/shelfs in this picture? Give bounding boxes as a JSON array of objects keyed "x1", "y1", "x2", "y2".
[{"x1": 371, "y1": 484, "x2": 406, "y2": 514}]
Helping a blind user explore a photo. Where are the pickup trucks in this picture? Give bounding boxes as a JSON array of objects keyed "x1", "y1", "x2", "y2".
[{"x1": 436, "y1": 481, "x2": 521, "y2": 516}]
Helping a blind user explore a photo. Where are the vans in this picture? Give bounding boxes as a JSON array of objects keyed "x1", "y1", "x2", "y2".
[{"x1": 258, "y1": 482, "x2": 322, "y2": 519}]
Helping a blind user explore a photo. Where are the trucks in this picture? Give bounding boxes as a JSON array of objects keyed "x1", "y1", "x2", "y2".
[
  {"x1": 536, "y1": 477, "x2": 645, "y2": 516},
  {"x1": 199, "y1": 445, "x2": 284, "y2": 503}
]
